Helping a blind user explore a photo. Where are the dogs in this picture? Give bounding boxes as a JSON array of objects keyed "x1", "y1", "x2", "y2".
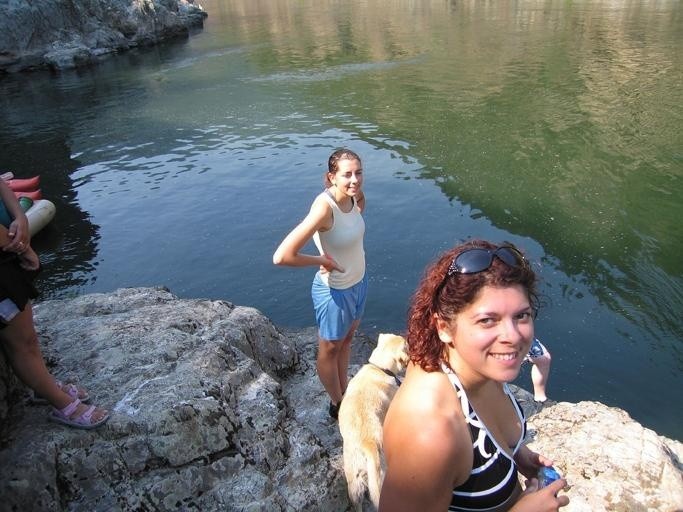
[{"x1": 335, "y1": 329, "x2": 414, "y2": 512}]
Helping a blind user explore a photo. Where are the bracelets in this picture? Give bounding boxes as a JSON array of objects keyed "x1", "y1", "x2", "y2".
[
  {"x1": 321, "y1": 253, "x2": 335, "y2": 270},
  {"x1": 356, "y1": 196, "x2": 366, "y2": 204}
]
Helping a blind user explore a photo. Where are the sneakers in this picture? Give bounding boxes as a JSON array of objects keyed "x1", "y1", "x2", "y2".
[{"x1": 329, "y1": 401, "x2": 340, "y2": 419}]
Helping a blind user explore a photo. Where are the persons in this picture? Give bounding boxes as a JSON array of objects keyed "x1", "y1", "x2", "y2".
[
  {"x1": 0, "y1": 174, "x2": 112, "y2": 431},
  {"x1": 519, "y1": 335, "x2": 557, "y2": 409},
  {"x1": 373, "y1": 238, "x2": 570, "y2": 512},
  {"x1": 272, "y1": 147, "x2": 369, "y2": 421}
]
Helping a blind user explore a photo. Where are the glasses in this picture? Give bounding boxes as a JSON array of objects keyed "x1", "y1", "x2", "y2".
[{"x1": 434, "y1": 248, "x2": 527, "y2": 298}]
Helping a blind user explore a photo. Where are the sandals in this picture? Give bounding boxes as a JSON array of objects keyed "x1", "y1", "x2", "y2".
[
  {"x1": 49, "y1": 400, "x2": 109, "y2": 428},
  {"x1": 31, "y1": 381, "x2": 89, "y2": 405}
]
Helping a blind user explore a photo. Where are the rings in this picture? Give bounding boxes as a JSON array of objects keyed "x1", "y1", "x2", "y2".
[{"x1": 18, "y1": 240, "x2": 24, "y2": 245}]
